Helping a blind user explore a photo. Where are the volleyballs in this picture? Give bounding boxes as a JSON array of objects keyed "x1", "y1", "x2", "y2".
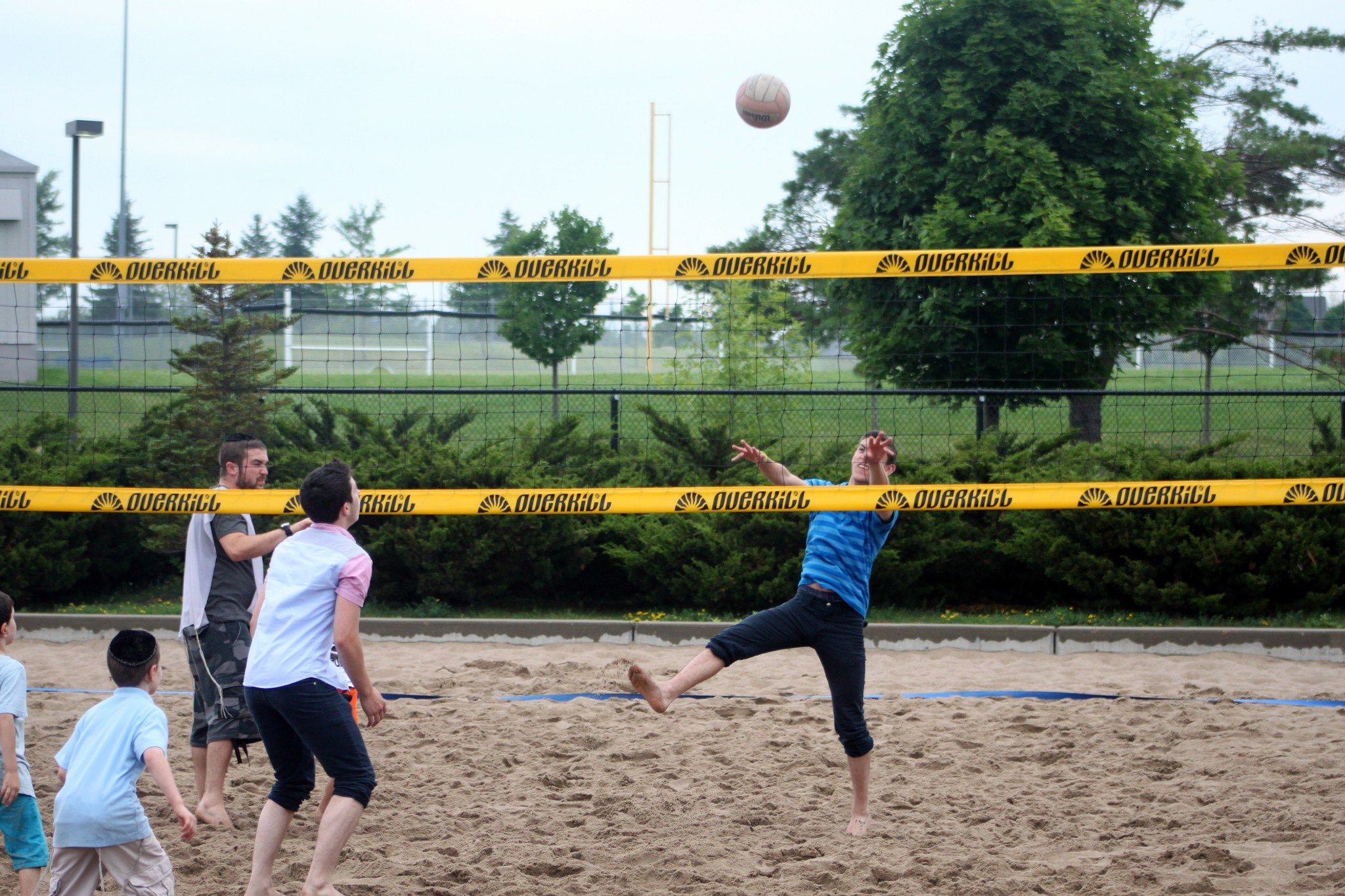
[{"x1": 735, "y1": 73, "x2": 791, "y2": 128}]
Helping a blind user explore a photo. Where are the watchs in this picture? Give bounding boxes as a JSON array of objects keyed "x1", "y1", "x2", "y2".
[{"x1": 279, "y1": 522, "x2": 293, "y2": 537}]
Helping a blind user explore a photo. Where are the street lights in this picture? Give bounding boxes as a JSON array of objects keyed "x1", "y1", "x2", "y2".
[
  {"x1": 165, "y1": 222, "x2": 179, "y2": 319},
  {"x1": 61, "y1": 118, "x2": 105, "y2": 455}
]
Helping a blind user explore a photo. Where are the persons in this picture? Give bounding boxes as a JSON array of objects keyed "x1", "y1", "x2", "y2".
[
  {"x1": 242, "y1": 464, "x2": 387, "y2": 896},
  {"x1": 0, "y1": 591, "x2": 48, "y2": 896},
  {"x1": 625, "y1": 430, "x2": 901, "y2": 838},
  {"x1": 175, "y1": 432, "x2": 313, "y2": 831},
  {"x1": 49, "y1": 629, "x2": 198, "y2": 895}
]
